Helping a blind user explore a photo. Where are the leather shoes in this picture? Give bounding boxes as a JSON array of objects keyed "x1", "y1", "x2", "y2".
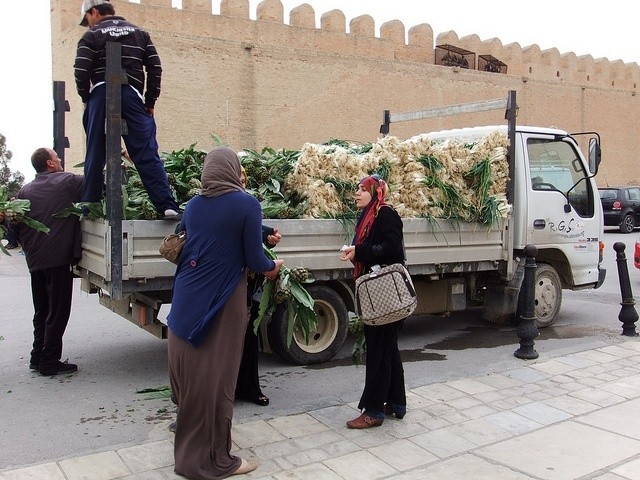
[
  {"x1": 385, "y1": 404, "x2": 406, "y2": 419},
  {"x1": 232, "y1": 458, "x2": 258, "y2": 474},
  {"x1": 347, "y1": 412, "x2": 384, "y2": 429},
  {"x1": 249, "y1": 395, "x2": 269, "y2": 406}
]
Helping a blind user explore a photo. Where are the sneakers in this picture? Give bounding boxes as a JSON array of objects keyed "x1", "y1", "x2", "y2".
[
  {"x1": 39, "y1": 358, "x2": 78, "y2": 376},
  {"x1": 29, "y1": 354, "x2": 42, "y2": 369}
]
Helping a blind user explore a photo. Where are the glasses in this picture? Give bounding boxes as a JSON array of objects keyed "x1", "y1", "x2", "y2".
[{"x1": 371, "y1": 174, "x2": 383, "y2": 187}]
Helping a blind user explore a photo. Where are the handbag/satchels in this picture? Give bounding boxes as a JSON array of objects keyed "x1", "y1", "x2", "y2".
[
  {"x1": 159, "y1": 231, "x2": 186, "y2": 265},
  {"x1": 354, "y1": 263, "x2": 418, "y2": 326}
]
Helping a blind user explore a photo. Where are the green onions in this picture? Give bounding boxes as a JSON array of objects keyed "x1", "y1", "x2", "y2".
[{"x1": 289, "y1": 131, "x2": 510, "y2": 248}]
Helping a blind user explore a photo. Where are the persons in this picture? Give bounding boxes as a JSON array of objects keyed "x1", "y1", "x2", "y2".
[
  {"x1": 339, "y1": 173, "x2": 406, "y2": 430},
  {"x1": 14, "y1": 148, "x2": 84, "y2": 375},
  {"x1": 164, "y1": 147, "x2": 259, "y2": 479},
  {"x1": 78, "y1": 0, "x2": 182, "y2": 220},
  {"x1": 238, "y1": 163, "x2": 282, "y2": 406}
]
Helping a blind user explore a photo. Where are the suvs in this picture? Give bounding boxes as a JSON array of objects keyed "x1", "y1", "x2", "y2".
[{"x1": 597, "y1": 180, "x2": 640, "y2": 233}]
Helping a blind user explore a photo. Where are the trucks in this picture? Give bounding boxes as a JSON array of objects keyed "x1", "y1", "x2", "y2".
[{"x1": 54, "y1": 42, "x2": 606, "y2": 365}]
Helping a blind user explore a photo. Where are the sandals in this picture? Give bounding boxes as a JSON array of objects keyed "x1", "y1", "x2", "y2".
[{"x1": 163, "y1": 210, "x2": 183, "y2": 220}]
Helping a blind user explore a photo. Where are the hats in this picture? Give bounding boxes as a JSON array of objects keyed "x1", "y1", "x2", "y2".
[{"x1": 78, "y1": 1, "x2": 111, "y2": 26}]
